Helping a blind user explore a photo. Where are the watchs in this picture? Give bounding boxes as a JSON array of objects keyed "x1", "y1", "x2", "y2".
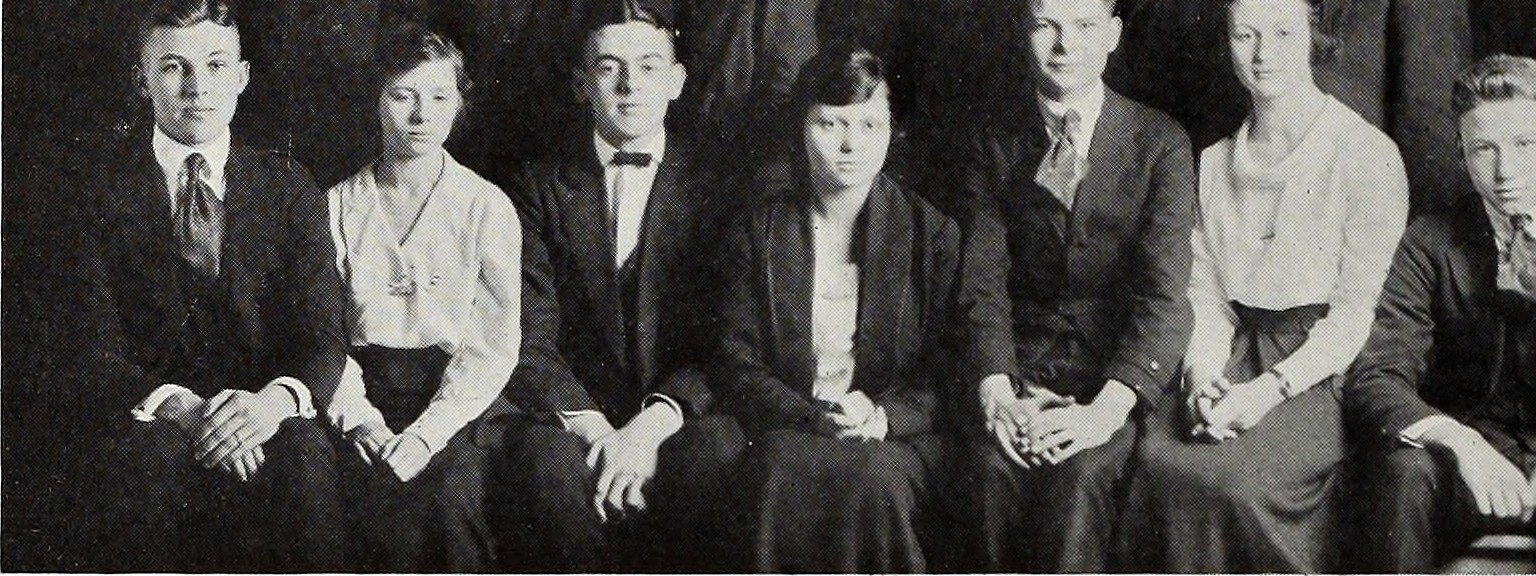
[{"x1": 1268, "y1": 367, "x2": 1293, "y2": 400}]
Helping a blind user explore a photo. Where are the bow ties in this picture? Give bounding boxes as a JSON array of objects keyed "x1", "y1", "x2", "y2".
[{"x1": 604, "y1": 151, "x2": 652, "y2": 169}]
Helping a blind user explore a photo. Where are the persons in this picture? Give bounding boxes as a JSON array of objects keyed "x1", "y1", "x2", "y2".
[
  {"x1": 1344, "y1": 53, "x2": 1536, "y2": 575},
  {"x1": 67, "y1": 0, "x2": 351, "y2": 574},
  {"x1": 1114, "y1": 0, "x2": 1410, "y2": 574},
  {"x1": 513, "y1": 0, "x2": 749, "y2": 574},
  {"x1": 325, "y1": 26, "x2": 524, "y2": 574},
  {"x1": 713, "y1": 48, "x2": 964, "y2": 574},
  {"x1": 947, "y1": 0, "x2": 1195, "y2": 574}
]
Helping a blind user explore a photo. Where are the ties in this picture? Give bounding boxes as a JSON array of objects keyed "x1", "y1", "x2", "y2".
[
  {"x1": 1034, "y1": 108, "x2": 1091, "y2": 210},
  {"x1": 175, "y1": 154, "x2": 223, "y2": 297},
  {"x1": 1497, "y1": 215, "x2": 1536, "y2": 294}
]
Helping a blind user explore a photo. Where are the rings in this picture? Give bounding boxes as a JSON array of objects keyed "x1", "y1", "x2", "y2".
[
  {"x1": 613, "y1": 480, "x2": 626, "y2": 488},
  {"x1": 233, "y1": 433, "x2": 242, "y2": 444}
]
